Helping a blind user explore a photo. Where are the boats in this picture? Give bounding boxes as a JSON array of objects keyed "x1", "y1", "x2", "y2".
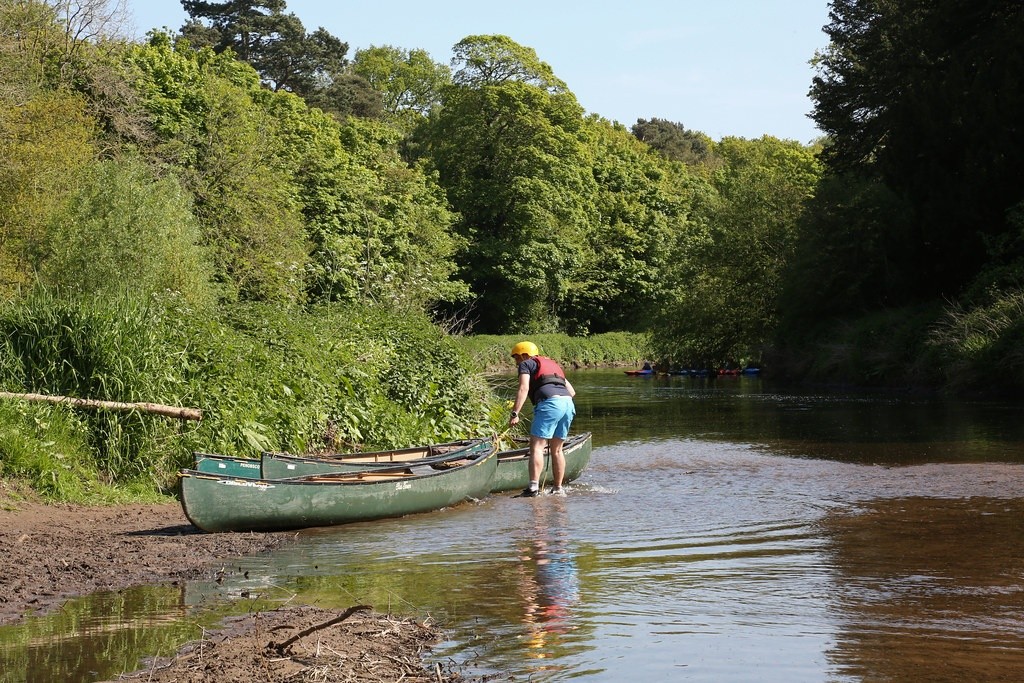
[
  {"x1": 260, "y1": 432, "x2": 592, "y2": 493},
  {"x1": 192, "y1": 437, "x2": 492, "y2": 479},
  {"x1": 670, "y1": 368, "x2": 760, "y2": 375},
  {"x1": 624, "y1": 369, "x2": 652, "y2": 375},
  {"x1": 175, "y1": 445, "x2": 499, "y2": 533}
]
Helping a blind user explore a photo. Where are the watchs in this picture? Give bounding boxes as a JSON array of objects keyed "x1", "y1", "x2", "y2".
[{"x1": 510, "y1": 410, "x2": 520, "y2": 418}]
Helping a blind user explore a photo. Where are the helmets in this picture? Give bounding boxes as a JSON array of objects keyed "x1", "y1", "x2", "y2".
[{"x1": 511, "y1": 342, "x2": 539, "y2": 358}]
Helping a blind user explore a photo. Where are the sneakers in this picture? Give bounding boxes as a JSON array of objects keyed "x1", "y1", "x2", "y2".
[
  {"x1": 513, "y1": 488, "x2": 540, "y2": 498},
  {"x1": 550, "y1": 489, "x2": 564, "y2": 494}
]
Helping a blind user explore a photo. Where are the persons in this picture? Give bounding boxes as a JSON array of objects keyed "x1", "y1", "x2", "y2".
[
  {"x1": 638, "y1": 357, "x2": 762, "y2": 371},
  {"x1": 509, "y1": 340, "x2": 577, "y2": 501}
]
